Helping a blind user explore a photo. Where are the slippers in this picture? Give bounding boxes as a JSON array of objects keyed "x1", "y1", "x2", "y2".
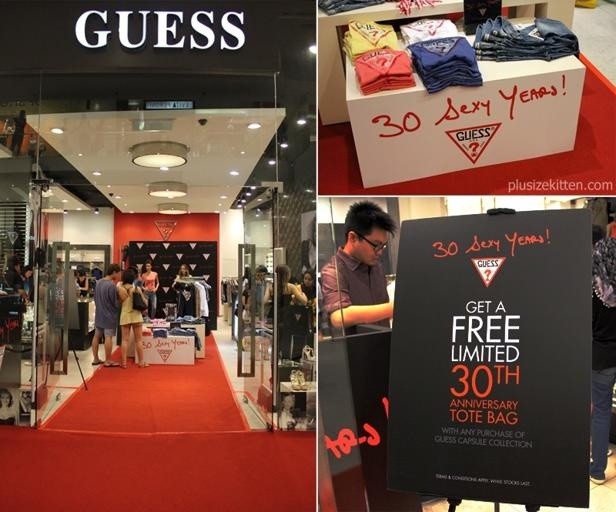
[
  {"x1": 92, "y1": 361, "x2": 105, "y2": 365},
  {"x1": 103, "y1": 363, "x2": 120, "y2": 367}
]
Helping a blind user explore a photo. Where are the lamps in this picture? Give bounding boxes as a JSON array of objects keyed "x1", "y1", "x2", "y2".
[
  {"x1": 158, "y1": 203, "x2": 189, "y2": 215},
  {"x1": 130, "y1": 142, "x2": 189, "y2": 168},
  {"x1": 176, "y1": 188, "x2": 177, "y2": 189},
  {"x1": 148, "y1": 182, "x2": 187, "y2": 198}
]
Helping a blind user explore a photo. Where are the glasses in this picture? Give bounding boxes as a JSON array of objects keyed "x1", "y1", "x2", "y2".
[{"x1": 356, "y1": 232, "x2": 391, "y2": 253}]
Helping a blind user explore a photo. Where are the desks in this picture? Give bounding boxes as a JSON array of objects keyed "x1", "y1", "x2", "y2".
[{"x1": 126, "y1": 323, "x2": 206, "y2": 358}]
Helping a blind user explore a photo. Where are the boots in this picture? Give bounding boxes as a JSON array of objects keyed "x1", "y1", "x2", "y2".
[
  {"x1": 254, "y1": 339, "x2": 260, "y2": 360},
  {"x1": 263, "y1": 339, "x2": 269, "y2": 360}
]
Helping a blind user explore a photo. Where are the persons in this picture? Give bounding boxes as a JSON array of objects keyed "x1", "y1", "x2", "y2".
[
  {"x1": 141, "y1": 259, "x2": 160, "y2": 319},
  {"x1": 320, "y1": 199, "x2": 395, "y2": 334},
  {"x1": 4, "y1": 248, "x2": 90, "y2": 361},
  {"x1": 92, "y1": 263, "x2": 120, "y2": 366},
  {"x1": 117, "y1": 269, "x2": 149, "y2": 368},
  {"x1": 588, "y1": 226, "x2": 616, "y2": 483},
  {"x1": 172, "y1": 264, "x2": 193, "y2": 304},
  {"x1": 234, "y1": 262, "x2": 316, "y2": 365}
]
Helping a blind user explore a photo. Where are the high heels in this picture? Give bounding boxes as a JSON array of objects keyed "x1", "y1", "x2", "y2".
[
  {"x1": 297, "y1": 370, "x2": 308, "y2": 390},
  {"x1": 302, "y1": 344, "x2": 315, "y2": 362},
  {"x1": 289, "y1": 370, "x2": 302, "y2": 390}
]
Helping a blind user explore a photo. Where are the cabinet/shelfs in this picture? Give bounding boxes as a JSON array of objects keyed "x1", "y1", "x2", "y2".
[{"x1": 318, "y1": 0, "x2": 575, "y2": 127}]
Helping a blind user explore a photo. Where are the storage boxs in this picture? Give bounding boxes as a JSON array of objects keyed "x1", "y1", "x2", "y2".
[
  {"x1": 345, "y1": 33, "x2": 586, "y2": 190},
  {"x1": 135, "y1": 335, "x2": 195, "y2": 365}
]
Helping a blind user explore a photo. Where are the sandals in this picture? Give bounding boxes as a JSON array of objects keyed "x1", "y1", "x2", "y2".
[
  {"x1": 120, "y1": 362, "x2": 126, "y2": 368},
  {"x1": 139, "y1": 362, "x2": 149, "y2": 368}
]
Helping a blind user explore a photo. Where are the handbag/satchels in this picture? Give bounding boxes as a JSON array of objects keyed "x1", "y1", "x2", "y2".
[{"x1": 132, "y1": 292, "x2": 148, "y2": 312}]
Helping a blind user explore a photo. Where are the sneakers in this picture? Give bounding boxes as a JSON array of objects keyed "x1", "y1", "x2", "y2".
[{"x1": 590, "y1": 473, "x2": 607, "y2": 485}]
